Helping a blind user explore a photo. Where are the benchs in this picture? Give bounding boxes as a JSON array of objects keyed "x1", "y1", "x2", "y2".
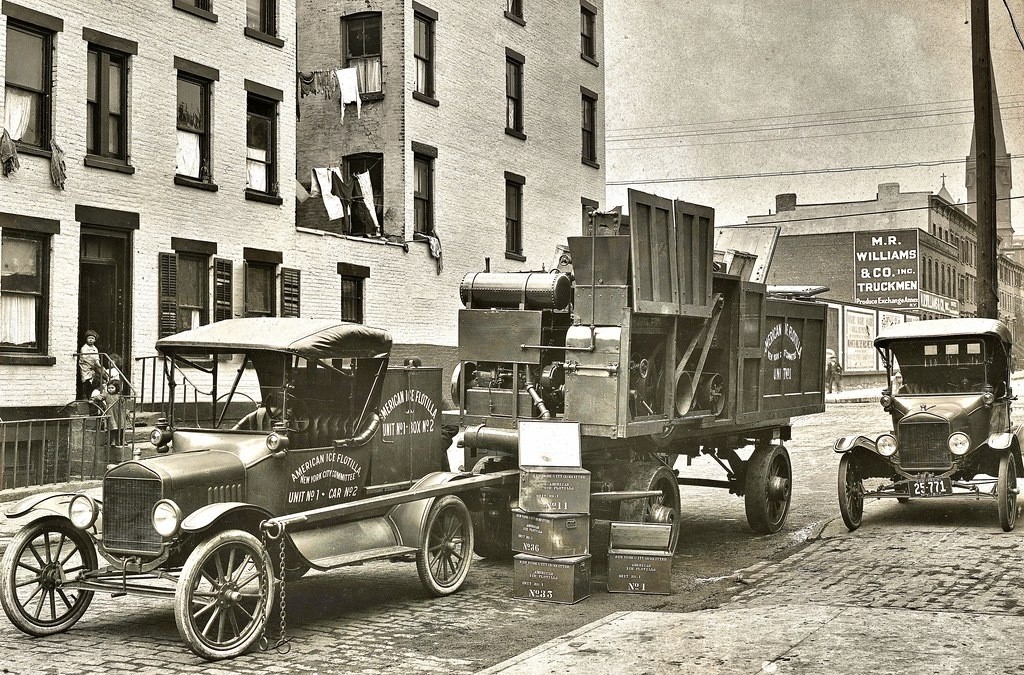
[
  {"x1": 230, "y1": 408, "x2": 379, "y2": 450},
  {"x1": 895, "y1": 368, "x2": 987, "y2": 394}
]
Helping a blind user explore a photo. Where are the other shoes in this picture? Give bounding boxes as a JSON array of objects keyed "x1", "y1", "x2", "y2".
[
  {"x1": 111, "y1": 441, "x2": 116, "y2": 446},
  {"x1": 837, "y1": 390, "x2": 841, "y2": 392},
  {"x1": 827, "y1": 391, "x2": 832, "y2": 394},
  {"x1": 123, "y1": 441, "x2": 127, "y2": 445}
]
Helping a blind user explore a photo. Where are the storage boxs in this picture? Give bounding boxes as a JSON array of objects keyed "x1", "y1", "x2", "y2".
[
  {"x1": 510, "y1": 509, "x2": 591, "y2": 560},
  {"x1": 519, "y1": 419, "x2": 593, "y2": 515},
  {"x1": 607, "y1": 522, "x2": 673, "y2": 594},
  {"x1": 512, "y1": 552, "x2": 591, "y2": 606}
]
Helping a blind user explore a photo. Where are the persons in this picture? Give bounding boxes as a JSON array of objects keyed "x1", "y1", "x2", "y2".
[
  {"x1": 89, "y1": 375, "x2": 106, "y2": 432},
  {"x1": 441, "y1": 393, "x2": 460, "y2": 473},
  {"x1": 79, "y1": 330, "x2": 101, "y2": 396},
  {"x1": 827, "y1": 356, "x2": 843, "y2": 393},
  {"x1": 102, "y1": 353, "x2": 123, "y2": 394},
  {"x1": 91, "y1": 379, "x2": 134, "y2": 447}
]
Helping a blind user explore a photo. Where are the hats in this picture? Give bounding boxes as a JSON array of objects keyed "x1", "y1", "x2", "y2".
[{"x1": 830, "y1": 355, "x2": 837, "y2": 357}]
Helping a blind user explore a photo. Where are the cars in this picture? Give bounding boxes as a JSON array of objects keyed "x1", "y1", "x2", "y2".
[
  {"x1": 836, "y1": 315, "x2": 1024, "y2": 532},
  {"x1": 0, "y1": 313, "x2": 489, "y2": 662}
]
